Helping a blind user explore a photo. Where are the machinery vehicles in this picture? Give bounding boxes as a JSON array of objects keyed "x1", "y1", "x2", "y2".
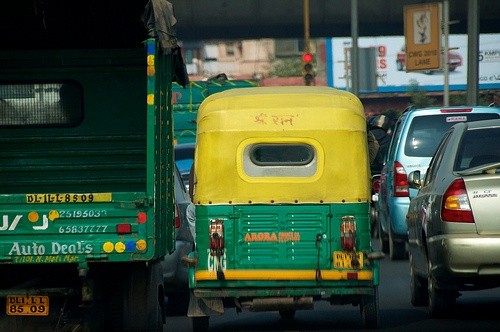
[{"x1": 172, "y1": 80, "x2": 256, "y2": 163}]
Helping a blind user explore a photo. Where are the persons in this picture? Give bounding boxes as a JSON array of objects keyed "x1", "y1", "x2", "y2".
[
  {"x1": 57, "y1": 84, "x2": 84, "y2": 124},
  {"x1": 185, "y1": 162, "x2": 194, "y2": 245},
  {"x1": 368, "y1": 112, "x2": 392, "y2": 177}
]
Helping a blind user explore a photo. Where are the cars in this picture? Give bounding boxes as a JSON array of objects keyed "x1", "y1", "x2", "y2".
[
  {"x1": 406, "y1": 118, "x2": 500, "y2": 318},
  {"x1": 396, "y1": 44, "x2": 462, "y2": 75},
  {"x1": 162, "y1": 162, "x2": 190, "y2": 314}
]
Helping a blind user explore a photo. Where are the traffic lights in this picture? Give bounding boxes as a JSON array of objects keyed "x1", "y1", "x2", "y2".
[{"x1": 300, "y1": 52, "x2": 317, "y2": 83}]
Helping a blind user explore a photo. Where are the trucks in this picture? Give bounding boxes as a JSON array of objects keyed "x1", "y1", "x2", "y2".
[{"x1": 1, "y1": 0, "x2": 178, "y2": 332}]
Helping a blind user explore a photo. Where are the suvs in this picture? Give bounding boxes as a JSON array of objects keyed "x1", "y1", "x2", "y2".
[{"x1": 378, "y1": 103, "x2": 500, "y2": 259}]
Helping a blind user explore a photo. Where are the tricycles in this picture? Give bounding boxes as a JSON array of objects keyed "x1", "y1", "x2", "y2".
[{"x1": 190, "y1": 86, "x2": 378, "y2": 329}]
